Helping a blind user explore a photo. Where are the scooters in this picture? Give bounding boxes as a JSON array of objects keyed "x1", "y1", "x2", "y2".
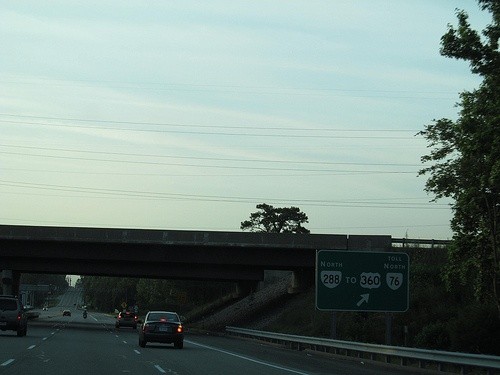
[{"x1": 82, "y1": 311, "x2": 88, "y2": 318}]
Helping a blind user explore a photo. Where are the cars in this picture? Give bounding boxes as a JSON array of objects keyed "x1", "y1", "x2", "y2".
[
  {"x1": 62, "y1": 309, "x2": 71, "y2": 317},
  {"x1": 24, "y1": 305, "x2": 41, "y2": 320},
  {"x1": 138, "y1": 311, "x2": 185, "y2": 350},
  {"x1": 114, "y1": 312, "x2": 138, "y2": 330}
]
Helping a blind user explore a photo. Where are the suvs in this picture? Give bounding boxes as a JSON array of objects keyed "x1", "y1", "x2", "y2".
[{"x1": 0, "y1": 295, "x2": 29, "y2": 337}]
